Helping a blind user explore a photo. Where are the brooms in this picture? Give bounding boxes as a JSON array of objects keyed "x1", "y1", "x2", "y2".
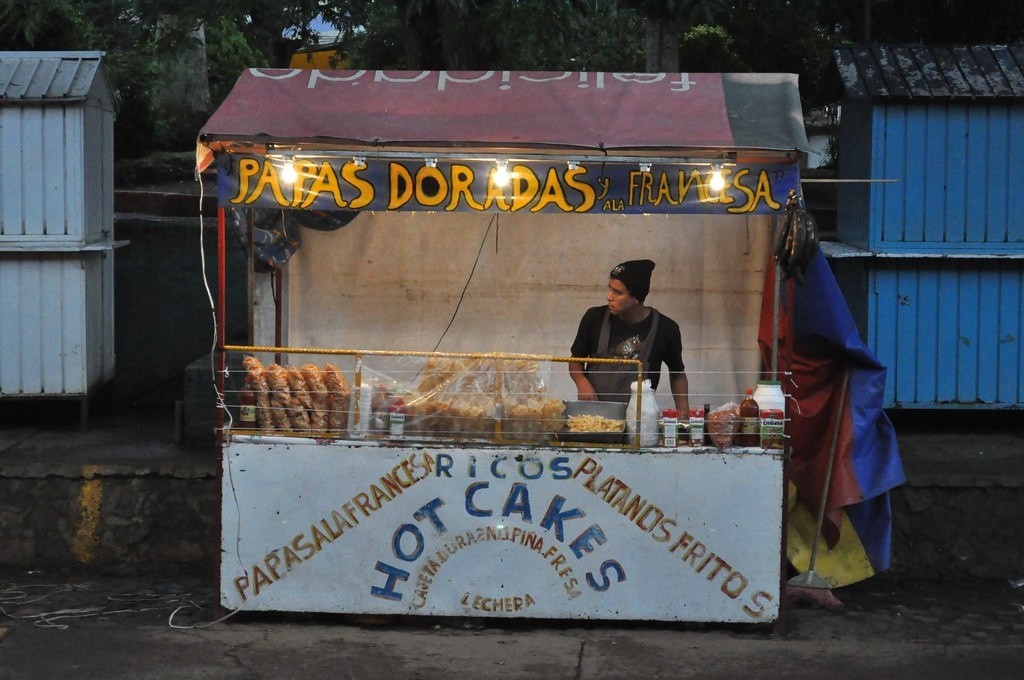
[{"x1": 782, "y1": 367, "x2": 851, "y2": 611}]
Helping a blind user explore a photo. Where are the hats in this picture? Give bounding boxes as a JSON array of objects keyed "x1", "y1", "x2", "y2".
[{"x1": 611, "y1": 260, "x2": 655, "y2": 302}]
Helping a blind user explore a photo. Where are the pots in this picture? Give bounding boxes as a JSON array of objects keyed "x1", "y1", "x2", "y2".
[{"x1": 560, "y1": 401, "x2": 628, "y2": 420}]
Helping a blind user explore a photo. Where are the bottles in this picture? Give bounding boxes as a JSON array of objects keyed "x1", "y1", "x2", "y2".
[
  {"x1": 626, "y1": 379, "x2": 660, "y2": 447},
  {"x1": 740, "y1": 389, "x2": 761, "y2": 446},
  {"x1": 687, "y1": 408, "x2": 705, "y2": 446},
  {"x1": 238, "y1": 377, "x2": 257, "y2": 428},
  {"x1": 753, "y1": 380, "x2": 785, "y2": 432},
  {"x1": 372, "y1": 384, "x2": 405, "y2": 436},
  {"x1": 662, "y1": 409, "x2": 679, "y2": 447}
]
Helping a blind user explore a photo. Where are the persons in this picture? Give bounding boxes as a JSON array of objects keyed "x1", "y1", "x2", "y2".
[{"x1": 568, "y1": 259, "x2": 690, "y2": 421}]
